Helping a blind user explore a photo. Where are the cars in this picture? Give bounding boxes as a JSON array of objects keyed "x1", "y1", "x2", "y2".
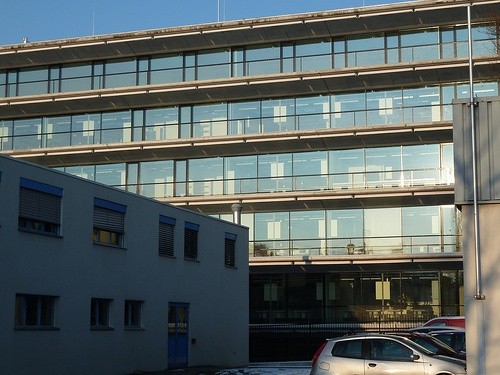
[{"x1": 385, "y1": 316, "x2": 467, "y2": 362}]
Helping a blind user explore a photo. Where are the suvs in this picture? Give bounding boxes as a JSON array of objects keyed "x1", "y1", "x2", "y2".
[{"x1": 313, "y1": 336, "x2": 467, "y2": 374}]
